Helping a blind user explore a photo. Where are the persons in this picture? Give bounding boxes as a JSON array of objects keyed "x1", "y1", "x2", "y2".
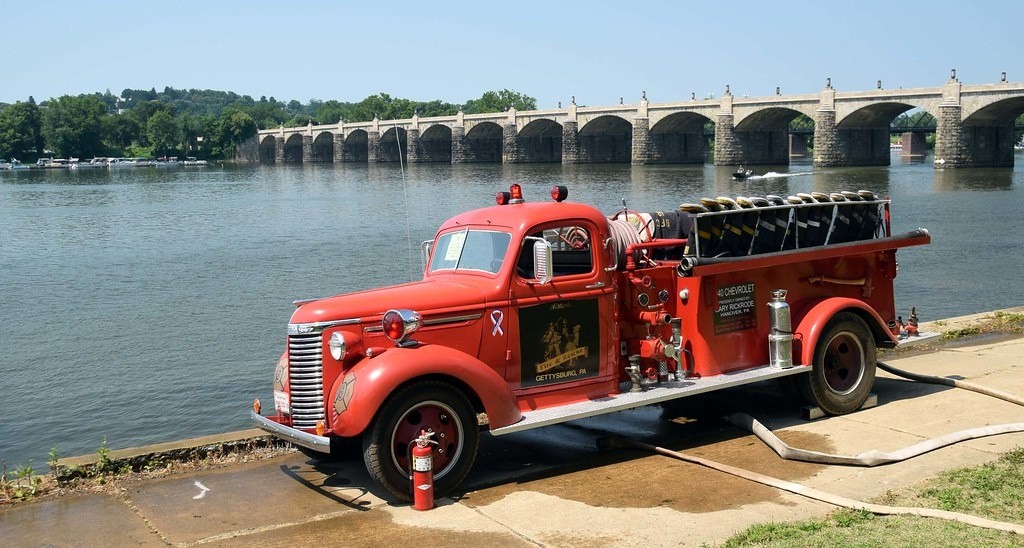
[{"x1": 737, "y1": 164, "x2": 751, "y2": 176}]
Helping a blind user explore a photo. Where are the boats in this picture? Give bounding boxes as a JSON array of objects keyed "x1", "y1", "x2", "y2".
[{"x1": 732, "y1": 173, "x2": 747, "y2": 179}]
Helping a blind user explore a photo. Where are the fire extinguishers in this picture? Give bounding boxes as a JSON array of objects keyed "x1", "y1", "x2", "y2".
[{"x1": 405, "y1": 429, "x2": 439, "y2": 510}]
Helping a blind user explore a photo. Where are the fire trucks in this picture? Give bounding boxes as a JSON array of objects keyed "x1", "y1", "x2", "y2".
[{"x1": 251, "y1": 182, "x2": 932, "y2": 505}]
